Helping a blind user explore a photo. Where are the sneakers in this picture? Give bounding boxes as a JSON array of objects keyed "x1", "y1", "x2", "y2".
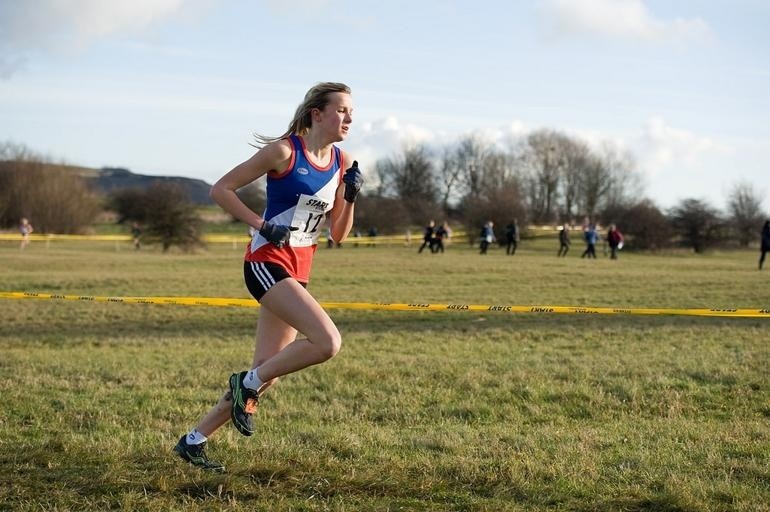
[
  {"x1": 230, "y1": 371, "x2": 259, "y2": 437},
  {"x1": 173, "y1": 433, "x2": 226, "y2": 474}
]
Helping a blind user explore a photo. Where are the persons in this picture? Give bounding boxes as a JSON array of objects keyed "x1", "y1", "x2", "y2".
[
  {"x1": 757, "y1": 219, "x2": 769, "y2": 270},
  {"x1": 171, "y1": 81, "x2": 365, "y2": 473},
  {"x1": 419, "y1": 218, "x2": 622, "y2": 258},
  {"x1": 129, "y1": 221, "x2": 144, "y2": 249},
  {"x1": 20, "y1": 217, "x2": 35, "y2": 248}
]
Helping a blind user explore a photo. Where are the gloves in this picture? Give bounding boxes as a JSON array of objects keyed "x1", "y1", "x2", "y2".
[
  {"x1": 260, "y1": 220, "x2": 299, "y2": 250},
  {"x1": 343, "y1": 160, "x2": 364, "y2": 203}
]
